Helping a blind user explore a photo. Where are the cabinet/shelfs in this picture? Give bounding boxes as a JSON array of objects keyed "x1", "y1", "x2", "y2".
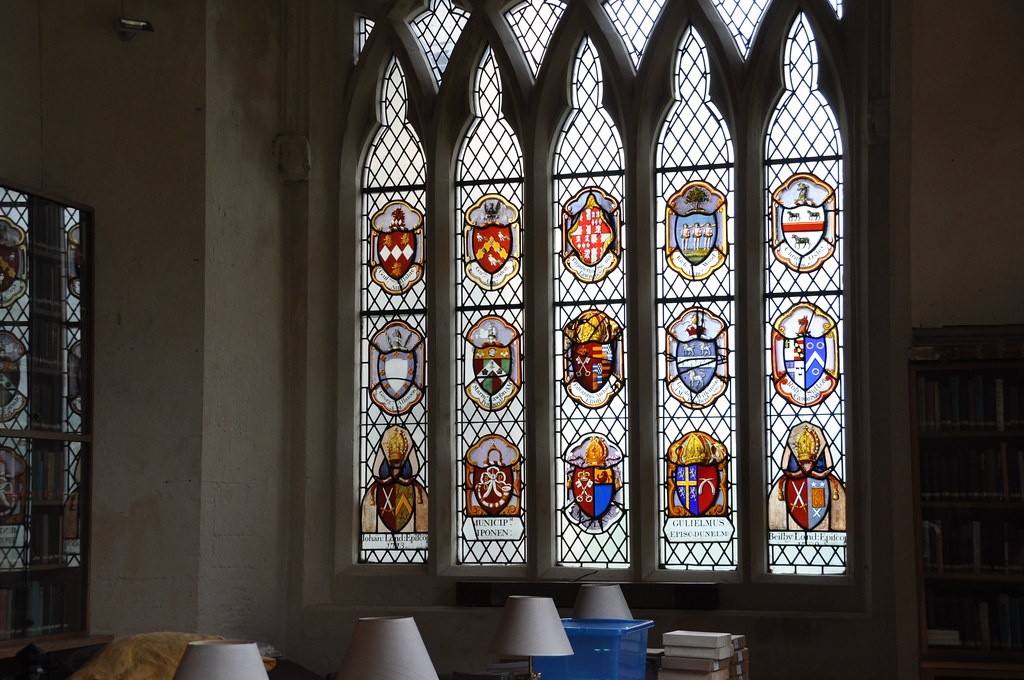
[
  {"x1": 910, "y1": 361, "x2": 1024, "y2": 680},
  {"x1": 0, "y1": 177, "x2": 117, "y2": 680}
]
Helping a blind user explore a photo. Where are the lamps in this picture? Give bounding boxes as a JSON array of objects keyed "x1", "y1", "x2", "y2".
[
  {"x1": 567, "y1": 584, "x2": 634, "y2": 620},
  {"x1": 172, "y1": 640, "x2": 270, "y2": 680},
  {"x1": 488, "y1": 596, "x2": 574, "y2": 680},
  {"x1": 117, "y1": 18, "x2": 156, "y2": 41},
  {"x1": 336, "y1": 616, "x2": 440, "y2": 680}
]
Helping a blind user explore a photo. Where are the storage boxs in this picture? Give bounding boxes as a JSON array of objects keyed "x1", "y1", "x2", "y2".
[
  {"x1": 657, "y1": 629, "x2": 749, "y2": 680},
  {"x1": 532, "y1": 617, "x2": 655, "y2": 680}
]
